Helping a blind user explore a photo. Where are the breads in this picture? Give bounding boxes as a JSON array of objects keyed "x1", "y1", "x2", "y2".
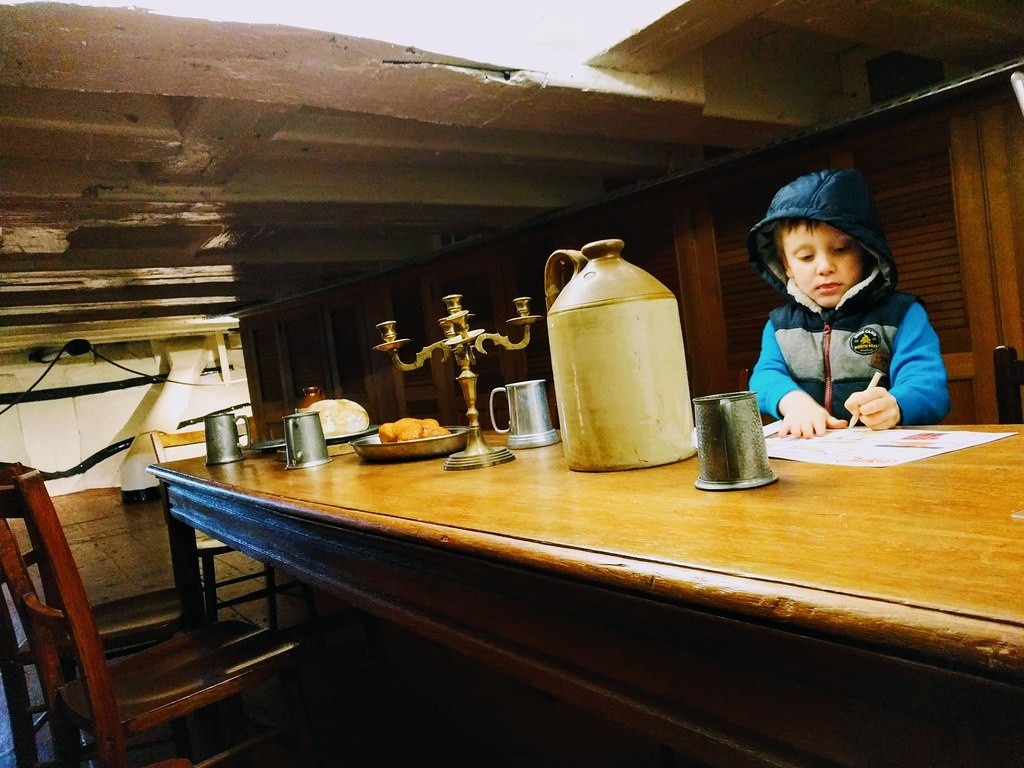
[
  {"x1": 379, "y1": 418, "x2": 453, "y2": 444},
  {"x1": 306, "y1": 399, "x2": 369, "y2": 437}
]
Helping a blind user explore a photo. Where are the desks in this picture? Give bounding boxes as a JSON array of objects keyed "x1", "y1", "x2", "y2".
[{"x1": 147, "y1": 424, "x2": 1024, "y2": 768}]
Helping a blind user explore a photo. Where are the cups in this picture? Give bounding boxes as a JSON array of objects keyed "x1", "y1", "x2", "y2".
[
  {"x1": 282, "y1": 408, "x2": 333, "y2": 470},
  {"x1": 692, "y1": 391, "x2": 779, "y2": 491},
  {"x1": 203, "y1": 413, "x2": 251, "y2": 466},
  {"x1": 489, "y1": 379, "x2": 560, "y2": 450}
]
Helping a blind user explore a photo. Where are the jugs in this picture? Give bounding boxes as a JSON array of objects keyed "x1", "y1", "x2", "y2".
[
  {"x1": 543, "y1": 238, "x2": 696, "y2": 473},
  {"x1": 295, "y1": 386, "x2": 325, "y2": 413}
]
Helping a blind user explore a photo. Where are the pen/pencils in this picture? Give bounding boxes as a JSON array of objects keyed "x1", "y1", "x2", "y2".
[{"x1": 849, "y1": 371, "x2": 883, "y2": 430}]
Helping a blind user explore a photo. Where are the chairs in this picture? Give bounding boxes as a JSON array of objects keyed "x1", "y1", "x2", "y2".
[
  {"x1": 1, "y1": 461, "x2": 194, "y2": 768},
  {"x1": 0, "y1": 464, "x2": 318, "y2": 768},
  {"x1": 150, "y1": 416, "x2": 318, "y2": 633}
]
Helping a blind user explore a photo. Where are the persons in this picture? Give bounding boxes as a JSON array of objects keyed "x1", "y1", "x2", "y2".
[{"x1": 744, "y1": 165, "x2": 953, "y2": 440}]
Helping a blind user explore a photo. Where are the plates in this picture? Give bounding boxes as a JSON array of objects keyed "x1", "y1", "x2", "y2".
[
  {"x1": 247, "y1": 439, "x2": 287, "y2": 454},
  {"x1": 347, "y1": 427, "x2": 470, "y2": 460},
  {"x1": 326, "y1": 424, "x2": 380, "y2": 446}
]
